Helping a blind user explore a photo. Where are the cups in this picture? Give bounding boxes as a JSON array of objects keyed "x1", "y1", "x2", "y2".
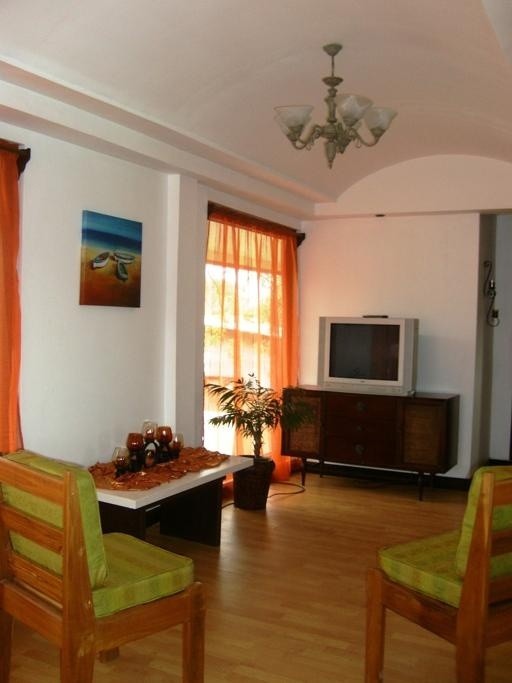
[{"x1": 112, "y1": 421, "x2": 184, "y2": 469}]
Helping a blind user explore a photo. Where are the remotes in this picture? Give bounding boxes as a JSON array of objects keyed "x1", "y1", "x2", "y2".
[{"x1": 363, "y1": 315, "x2": 388, "y2": 318}]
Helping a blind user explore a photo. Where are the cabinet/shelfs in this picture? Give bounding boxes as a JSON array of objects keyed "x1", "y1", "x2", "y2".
[{"x1": 281, "y1": 383, "x2": 460, "y2": 503}]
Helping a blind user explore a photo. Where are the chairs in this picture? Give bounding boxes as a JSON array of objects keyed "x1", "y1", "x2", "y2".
[
  {"x1": 364, "y1": 464, "x2": 511, "y2": 683},
  {"x1": 0, "y1": 449, "x2": 206, "y2": 683}
]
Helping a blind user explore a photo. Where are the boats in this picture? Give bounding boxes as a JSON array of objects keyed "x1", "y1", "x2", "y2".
[{"x1": 92, "y1": 250, "x2": 136, "y2": 280}]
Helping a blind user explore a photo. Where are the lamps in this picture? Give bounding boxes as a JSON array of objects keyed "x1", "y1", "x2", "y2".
[
  {"x1": 482, "y1": 261, "x2": 500, "y2": 327},
  {"x1": 274, "y1": 42, "x2": 397, "y2": 169}
]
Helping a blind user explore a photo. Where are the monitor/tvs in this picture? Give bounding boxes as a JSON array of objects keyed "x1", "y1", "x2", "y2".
[{"x1": 317, "y1": 316, "x2": 420, "y2": 397}]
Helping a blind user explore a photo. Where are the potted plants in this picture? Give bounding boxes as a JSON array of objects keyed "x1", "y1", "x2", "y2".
[{"x1": 202, "y1": 373, "x2": 317, "y2": 511}]
